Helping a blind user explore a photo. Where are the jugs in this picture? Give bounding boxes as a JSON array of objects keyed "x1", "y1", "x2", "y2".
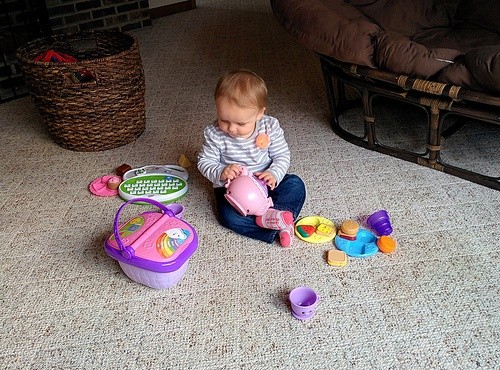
[{"x1": 224, "y1": 166, "x2": 274, "y2": 217}]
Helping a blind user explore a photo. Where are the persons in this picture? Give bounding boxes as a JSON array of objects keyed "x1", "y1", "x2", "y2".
[{"x1": 198, "y1": 69, "x2": 306, "y2": 248}]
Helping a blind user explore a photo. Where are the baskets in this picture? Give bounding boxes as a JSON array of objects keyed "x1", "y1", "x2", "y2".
[{"x1": 15, "y1": 29, "x2": 146, "y2": 152}]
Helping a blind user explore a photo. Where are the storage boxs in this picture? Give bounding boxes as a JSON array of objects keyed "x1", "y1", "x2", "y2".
[{"x1": 104, "y1": 199, "x2": 199, "y2": 289}]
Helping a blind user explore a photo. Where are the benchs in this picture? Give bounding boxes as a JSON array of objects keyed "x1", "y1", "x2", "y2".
[{"x1": 269, "y1": 0, "x2": 500, "y2": 190}]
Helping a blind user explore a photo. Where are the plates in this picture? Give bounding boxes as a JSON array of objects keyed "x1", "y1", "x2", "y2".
[{"x1": 295, "y1": 216, "x2": 336, "y2": 243}]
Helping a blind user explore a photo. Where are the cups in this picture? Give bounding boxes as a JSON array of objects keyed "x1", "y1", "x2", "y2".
[
  {"x1": 289, "y1": 287, "x2": 320, "y2": 317},
  {"x1": 367, "y1": 210, "x2": 393, "y2": 236}
]
